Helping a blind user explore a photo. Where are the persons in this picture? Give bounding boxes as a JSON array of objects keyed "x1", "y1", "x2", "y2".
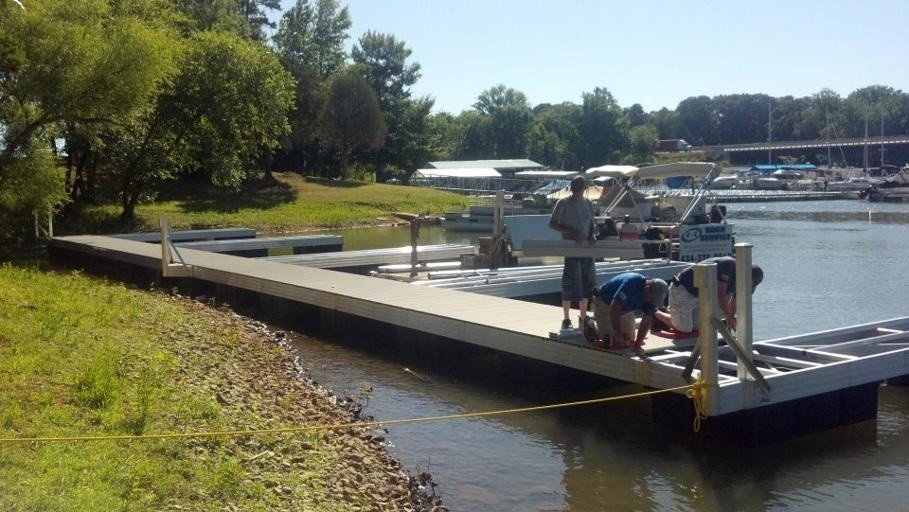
[
  {"x1": 621, "y1": 215, "x2": 638, "y2": 231},
  {"x1": 583, "y1": 272, "x2": 669, "y2": 355},
  {"x1": 548, "y1": 175, "x2": 597, "y2": 330},
  {"x1": 653, "y1": 256, "x2": 763, "y2": 333}
]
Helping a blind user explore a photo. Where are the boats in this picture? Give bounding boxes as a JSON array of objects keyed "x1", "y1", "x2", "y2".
[{"x1": 711, "y1": 160, "x2": 909, "y2": 202}]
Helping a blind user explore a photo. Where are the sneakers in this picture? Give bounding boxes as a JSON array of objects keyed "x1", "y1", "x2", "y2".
[
  {"x1": 651, "y1": 311, "x2": 663, "y2": 332},
  {"x1": 561, "y1": 315, "x2": 596, "y2": 342}
]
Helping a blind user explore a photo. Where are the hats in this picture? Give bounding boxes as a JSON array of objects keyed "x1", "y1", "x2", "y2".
[
  {"x1": 651, "y1": 278, "x2": 668, "y2": 308},
  {"x1": 752, "y1": 265, "x2": 763, "y2": 294},
  {"x1": 571, "y1": 175, "x2": 586, "y2": 191}
]
